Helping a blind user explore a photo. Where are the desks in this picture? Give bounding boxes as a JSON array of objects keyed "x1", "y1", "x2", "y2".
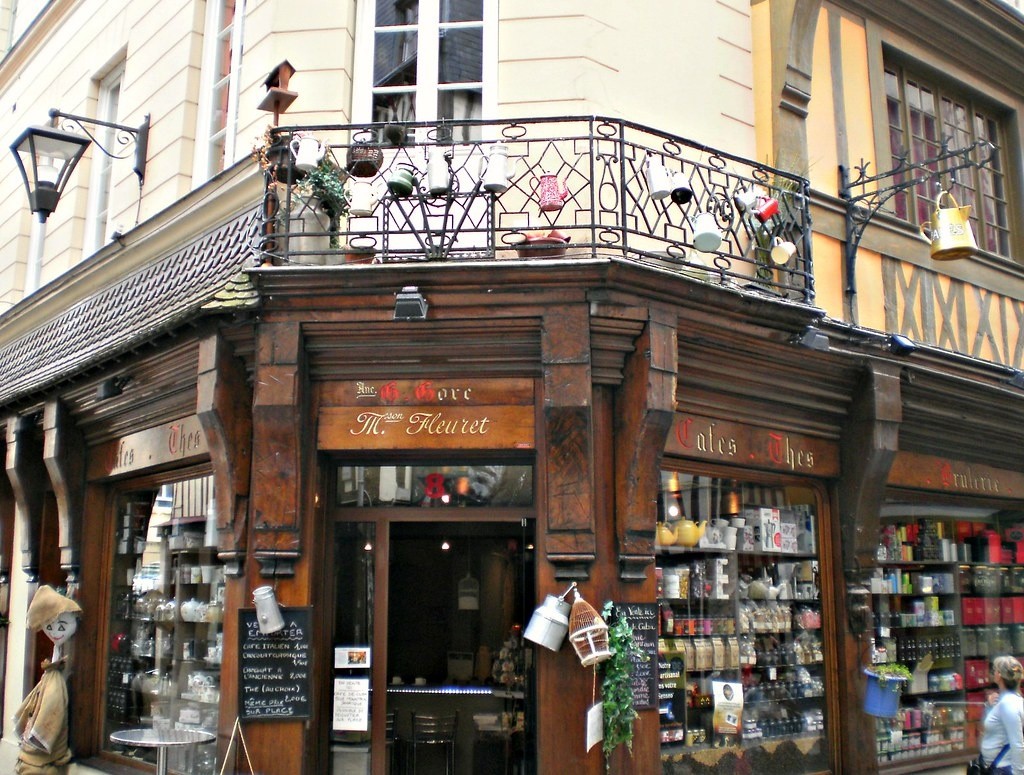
[{"x1": 110, "y1": 729, "x2": 220, "y2": 775}]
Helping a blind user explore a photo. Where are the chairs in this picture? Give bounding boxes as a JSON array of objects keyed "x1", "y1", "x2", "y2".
[
  {"x1": 403, "y1": 710, "x2": 460, "y2": 775},
  {"x1": 384, "y1": 707, "x2": 400, "y2": 775}
]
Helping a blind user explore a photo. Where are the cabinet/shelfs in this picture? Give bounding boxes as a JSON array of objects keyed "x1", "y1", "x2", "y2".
[
  {"x1": 867, "y1": 559, "x2": 1024, "y2": 775},
  {"x1": 656, "y1": 546, "x2": 831, "y2": 775},
  {"x1": 104, "y1": 516, "x2": 225, "y2": 775}
]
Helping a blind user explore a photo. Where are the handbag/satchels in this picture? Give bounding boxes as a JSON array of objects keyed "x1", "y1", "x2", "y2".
[{"x1": 967, "y1": 754, "x2": 997, "y2": 775}]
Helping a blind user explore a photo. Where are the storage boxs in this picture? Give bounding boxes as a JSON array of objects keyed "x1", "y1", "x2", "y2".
[{"x1": 654, "y1": 507, "x2": 1024, "y2": 749}]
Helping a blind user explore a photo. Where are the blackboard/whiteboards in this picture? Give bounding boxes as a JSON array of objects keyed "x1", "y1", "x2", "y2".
[
  {"x1": 609, "y1": 602, "x2": 660, "y2": 712},
  {"x1": 237, "y1": 606, "x2": 316, "y2": 723},
  {"x1": 657, "y1": 650, "x2": 688, "y2": 723}
]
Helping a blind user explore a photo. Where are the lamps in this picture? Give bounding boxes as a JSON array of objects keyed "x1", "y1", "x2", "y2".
[
  {"x1": 94, "y1": 375, "x2": 134, "y2": 402},
  {"x1": 1009, "y1": 367, "x2": 1024, "y2": 390},
  {"x1": 391, "y1": 284, "x2": 429, "y2": 323},
  {"x1": 789, "y1": 325, "x2": 830, "y2": 353},
  {"x1": 8, "y1": 107, "x2": 151, "y2": 220},
  {"x1": 889, "y1": 333, "x2": 920, "y2": 357}
]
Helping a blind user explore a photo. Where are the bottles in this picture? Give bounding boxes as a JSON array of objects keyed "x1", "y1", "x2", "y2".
[
  {"x1": 900, "y1": 634, "x2": 961, "y2": 661},
  {"x1": 660, "y1": 722, "x2": 706, "y2": 750},
  {"x1": 875, "y1": 701, "x2": 965, "y2": 763}
]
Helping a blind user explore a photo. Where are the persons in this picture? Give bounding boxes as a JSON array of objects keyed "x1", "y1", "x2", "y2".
[{"x1": 969, "y1": 654, "x2": 1024, "y2": 775}]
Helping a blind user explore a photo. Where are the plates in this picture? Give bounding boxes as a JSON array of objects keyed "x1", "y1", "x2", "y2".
[
  {"x1": 412, "y1": 684, "x2": 427, "y2": 686},
  {"x1": 388, "y1": 682, "x2": 404, "y2": 685}
]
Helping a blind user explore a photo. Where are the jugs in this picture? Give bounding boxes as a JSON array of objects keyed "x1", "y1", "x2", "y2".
[
  {"x1": 180, "y1": 598, "x2": 199, "y2": 621},
  {"x1": 344, "y1": 179, "x2": 386, "y2": 215},
  {"x1": 290, "y1": 135, "x2": 327, "y2": 171},
  {"x1": 386, "y1": 161, "x2": 424, "y2": 197},
  {"x1": 528, "y1": 171, "x2": 569, "y2": 211},
  {"x1": 187, "y1": 671, "x2": 214, "y2": 692},
  {"x1": 478, "y1": 141, "x2": 523, "y2": 194},
  {"x1": 732, "y1": 186, "x2": 761, "y2": 214},
  {"x1": 771, "y1": 237, "x2": 797, "y2": 264},
  {"x1": 687, "y1": 211, "x2": 722, "y2": 253},
  {"x1": 919, "y1": 190, "x2": 979, "y2": 260},
  {"x1": 754, "y1": 198, "x2": 781, "y2": 223}
]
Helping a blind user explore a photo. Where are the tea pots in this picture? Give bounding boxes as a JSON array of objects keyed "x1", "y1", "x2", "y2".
[
  {"x1": 654, "y1": 521, "x2": 679, "y2": 547},
  {"x1": 670, "y1": 517, "x2": 708, "y2": 547}
]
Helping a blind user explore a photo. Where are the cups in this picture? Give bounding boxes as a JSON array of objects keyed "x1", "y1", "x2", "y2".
[
  {"x1": 180, "y1": 561, "x2": 217, "y2": 583},
  {"x1": 152, "y1": 716, "x2": 170, "y2": 735},
  {"x1": 393, "y1": 677, "x2": 401, "y2": 684},
  {"x1": 183, "y1": 632, "x2": 222, "y2": 663},
  {"x1": 414, "y1": 678, "x2": 426, "y2": 684}
]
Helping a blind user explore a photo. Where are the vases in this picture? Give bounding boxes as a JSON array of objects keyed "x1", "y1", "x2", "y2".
[
  {"x1": 516, "y1": 237, "x2": 567, "y2": 261},
  {"x1": 345, "y1": 245, "x2": 377, "y2": 265}
]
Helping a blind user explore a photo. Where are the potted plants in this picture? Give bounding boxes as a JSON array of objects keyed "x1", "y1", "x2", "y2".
[
  {"x1": 863, "y1": 661, "x2": 914, "y2": 719},
  {"x1": 275, "y1": 151, "x2": 353, "y2": 265}
]
